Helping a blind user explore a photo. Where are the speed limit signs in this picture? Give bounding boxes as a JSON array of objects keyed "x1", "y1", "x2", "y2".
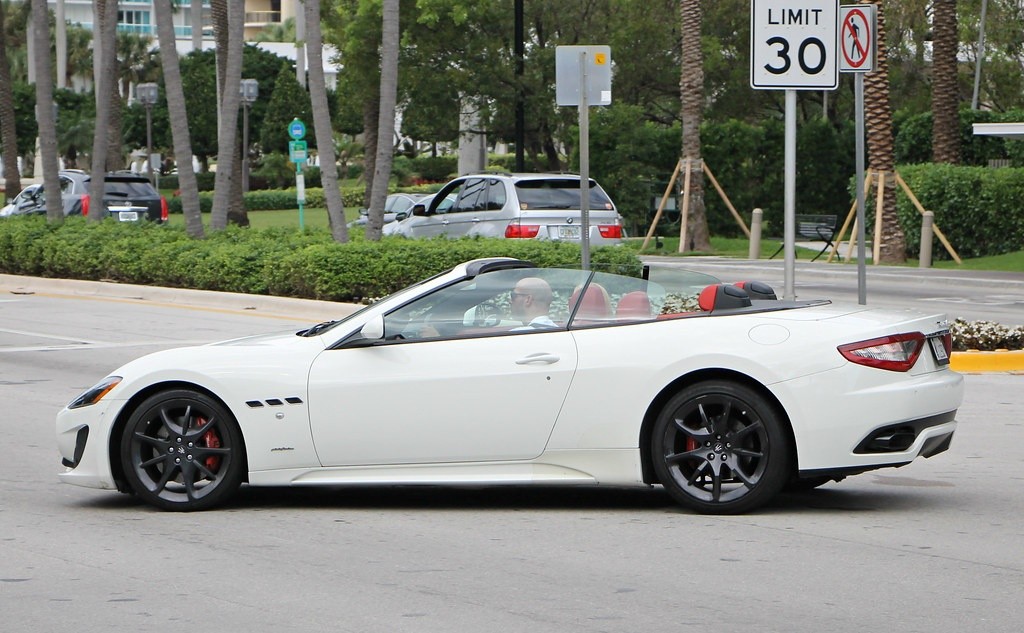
[{"x1": 750, "y1": 0, "x2": 839, "y2": 90}]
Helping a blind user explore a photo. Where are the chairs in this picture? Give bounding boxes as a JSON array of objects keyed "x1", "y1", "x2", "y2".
[
  {"x1": 733, "y1": 281, "x2": 746, "y2": 290},
  {"x1": 616, "y1": 290, "x2": 651, "y2": 320},
  {"x1": 657, "y1": 283, "x2": 718, "y2": 317},
  {"x1": 568, "y1": 283, "x2": 612, "y2": 325}
]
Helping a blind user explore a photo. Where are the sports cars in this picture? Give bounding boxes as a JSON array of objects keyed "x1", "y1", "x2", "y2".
[{"x1": 56, "y1": 254, "x2": 959, "y2": 514}]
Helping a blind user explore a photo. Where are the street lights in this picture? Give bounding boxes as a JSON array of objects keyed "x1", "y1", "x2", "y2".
[
  {"x1": 137, "y1": 81, "x2": 159, "y2": 184},
  {"x1": 238, "y1": 77, "x2": 258, "y2": 190}
]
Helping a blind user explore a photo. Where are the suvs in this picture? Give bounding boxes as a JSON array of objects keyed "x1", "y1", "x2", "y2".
[
  {"x1": 10, "y1": 169, "x2": 168, "y2": 227},
  {"x1": 380, "y1": 170, "x2": 626, "y2": 251}
]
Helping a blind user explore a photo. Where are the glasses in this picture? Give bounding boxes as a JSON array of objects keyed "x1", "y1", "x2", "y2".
[{"x1": 510, "y1": 292, "x2": 532, "y2": 299}]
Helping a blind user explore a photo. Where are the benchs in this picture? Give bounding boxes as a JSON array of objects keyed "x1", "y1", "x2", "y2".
[{"x1": 770, "y1": 214, "x2": 843, "y2": 263}]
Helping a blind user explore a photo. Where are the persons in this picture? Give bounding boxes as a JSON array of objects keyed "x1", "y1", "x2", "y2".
[
  {"x1": 308, "y1": 140, "x2": 413, "y2": 166},
  {"x1": 63, "y1": 143, "x2": 200, "y2": 174},
  {"x1": 416, "y1": 277, "x2": 559, "y2": 337}
]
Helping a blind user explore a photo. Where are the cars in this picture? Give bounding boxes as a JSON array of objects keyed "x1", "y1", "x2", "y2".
[
  {"x1": 0, "y1": 184, "x2": 41, "y2": 217},
  {"x1": 346, "y1": 191, "x2": 463, "y2": 228}
]
